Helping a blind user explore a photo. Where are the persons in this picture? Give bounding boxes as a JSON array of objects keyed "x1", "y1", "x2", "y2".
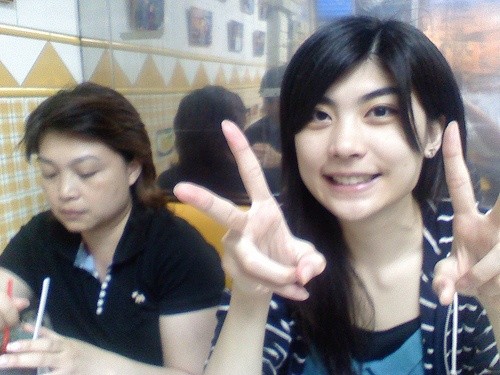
[
  {"x1": 245, "y1": 65, "x2": 287, "y2": 194},
  {"x1": 173, "y1": 17, "x2": 500, "y2": 375},
  {"x1": 0, "y1": 81, "x2": 227, "y2": 375},
  {"x1": 155, "y1": 85, "x2": 292, "y2": 207},
  {"x1": 463, "y1": 102, "x2": 499, "y2": 211}
]
